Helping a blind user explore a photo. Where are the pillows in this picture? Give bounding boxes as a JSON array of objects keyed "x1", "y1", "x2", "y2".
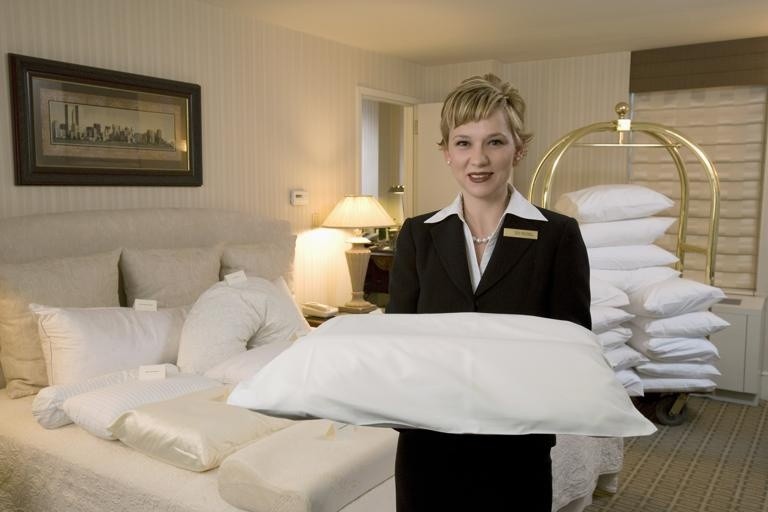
[
  {"x1": 632, "y1": 360, "x2": 723, "y2": 380},
  {"x1": 214, "y1": 235, "x2": 294, "y2": 299},
  {"x1": 585, "y1": 243, "x2": 681, "y2": 271},
  {"x1": 31, "y1": 363, "x2": 181, "y2": 430},
  {"x1": 589, "y1": 306, "x2": 636, "y2": 336},
  {"x1": 201, "y1": 338, "x2": 301, "y2": 385},
  {"x1": 589, "y1": 266, "x2": 683, "y2": 296},
  {"x1": 216, "y1": 421, "x2": 400, "y2": 510},
  {"x1": 0, "y1": 251, "x2": 124, "y2": 400},
  {"x1": 621, "y1": 277, "x2": 728, "y2": 319},
  {"x1": 227, "y1": 311, "x2": 658, "y2": 439},
  {"x1": 622, "y1": 322, "x2": 722, "y2": 364},
  {"x1": 590, "y1": 278, "x2": 630, "y2": 308},
  {"x1": 636, "y1": 372, "x2": 717, "y2": 392},
  {"x1": 119, "y1": 247, "x2": 224, "y2": 308},
  {"x1": 616, "y1": 368, "x2": 645, "y2": 398},
  {"x1": 31, "y1": 298, "x2": 190, "y2": 387},
  {"x1": 577, "y1": 215, "x2": 678, "y2": 248},
  {"x1": 106, "y1": 387, "x2": 296, "y2": 474},
  {"x1": 598, "y1": 324, "x2": 633, "y2": 353},
  {"x1": 175, "y1": 269, "x2": 312, "y2": 373},
  {"x1": 602, "y1": 343, "x2": 651, "y2": 372},
  {"x1": 61, "y1": 379, "x2": 226, "y2": 443},
  {"x1": 629, "y1": 310, "x2": 733, "y2": 339},
  {"x1": 555, "y1": 183, "x2": 675, "y2": 223}
]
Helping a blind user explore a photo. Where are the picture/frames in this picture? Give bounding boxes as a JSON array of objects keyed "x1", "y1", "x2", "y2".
[{"x1": 6, "y1": 51, "x2": 204, "y2": 188}]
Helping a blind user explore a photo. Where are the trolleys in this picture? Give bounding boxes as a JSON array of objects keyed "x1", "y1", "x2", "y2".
[{"x1": 527, "y1": 102, "x2": 720, "y2": 426}]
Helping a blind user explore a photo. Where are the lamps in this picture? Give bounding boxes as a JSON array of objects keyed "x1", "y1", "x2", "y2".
[{"x1": 318, "y1": 194, "x2": 397, "y2": 314}]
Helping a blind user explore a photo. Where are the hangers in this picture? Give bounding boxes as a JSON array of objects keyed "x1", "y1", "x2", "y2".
[{"x1": 631, "y1": 41, "x2": 764, "y2": 297}]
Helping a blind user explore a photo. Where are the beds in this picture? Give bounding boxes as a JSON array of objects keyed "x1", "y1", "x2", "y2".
[{"x1": 0, "y1": 206, "x2": 625, "y2": 512}]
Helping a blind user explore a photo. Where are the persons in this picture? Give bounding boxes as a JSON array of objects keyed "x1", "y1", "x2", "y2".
[{"x1": 385, "y1": 73, "x2": 592, "y2": 512}]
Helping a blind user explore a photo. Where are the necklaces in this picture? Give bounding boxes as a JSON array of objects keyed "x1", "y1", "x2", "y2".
[{"x1": 468, "y1": 234, "x2": 495, "y2": 243}]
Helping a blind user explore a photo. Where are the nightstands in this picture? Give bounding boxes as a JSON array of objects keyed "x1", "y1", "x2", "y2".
[{"x1": 305, "y1": 308, "x2": 351, "y2": 327}]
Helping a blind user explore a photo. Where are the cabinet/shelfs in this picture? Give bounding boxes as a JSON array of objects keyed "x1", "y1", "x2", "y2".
[{"x1": 710, "y1": 294, "x2": 766, "y2": 407}]
